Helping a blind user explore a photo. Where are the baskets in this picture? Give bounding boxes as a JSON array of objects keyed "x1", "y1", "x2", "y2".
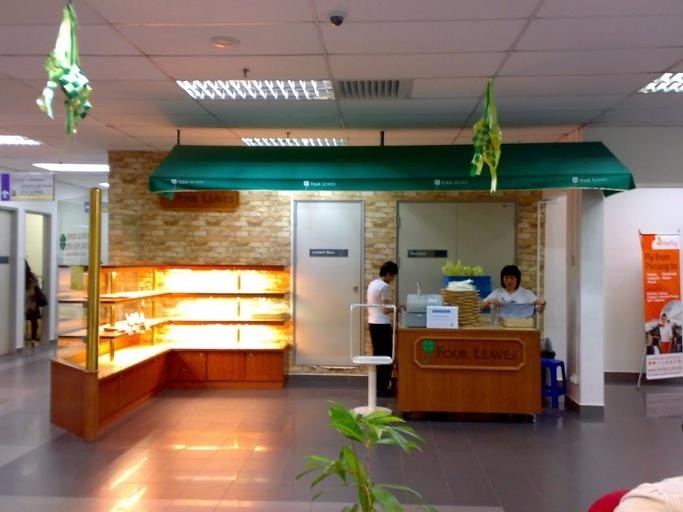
[{"x1": 438, "y1": 288, "x2": 480, "y2": 325}]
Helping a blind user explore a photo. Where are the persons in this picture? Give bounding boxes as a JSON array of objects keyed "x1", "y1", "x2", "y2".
[
  {"x1": 25, "y1": 259, "x2": 47, "y2": 348},
  {"x1": 644, "y1": 310, "x2": 682, "y2": 357},
  {"x1": 363, "y1": 260, "x2": 407, "y2": 403},
  {"x1": 473, "y1": 263, "x2": 546, "y2": 323}
]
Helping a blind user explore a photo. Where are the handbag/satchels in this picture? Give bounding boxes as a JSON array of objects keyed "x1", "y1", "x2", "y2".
[{"x1": 33, "y1": 285, "x2": 48, "y2": 307}]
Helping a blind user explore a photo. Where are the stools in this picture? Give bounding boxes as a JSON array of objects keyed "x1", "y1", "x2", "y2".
[{"x1": 541, "y1": 357, "x2": 567, "y2": 411}]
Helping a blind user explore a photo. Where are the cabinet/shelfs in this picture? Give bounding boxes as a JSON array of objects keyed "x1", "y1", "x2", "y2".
[{"x1": 46, "y1": 261, "x2": 296, "y2": 442}]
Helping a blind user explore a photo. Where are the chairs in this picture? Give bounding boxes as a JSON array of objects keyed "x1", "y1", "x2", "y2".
[{"x1": 588, "y1": 487, "x2": 638, "y2": 512}]
